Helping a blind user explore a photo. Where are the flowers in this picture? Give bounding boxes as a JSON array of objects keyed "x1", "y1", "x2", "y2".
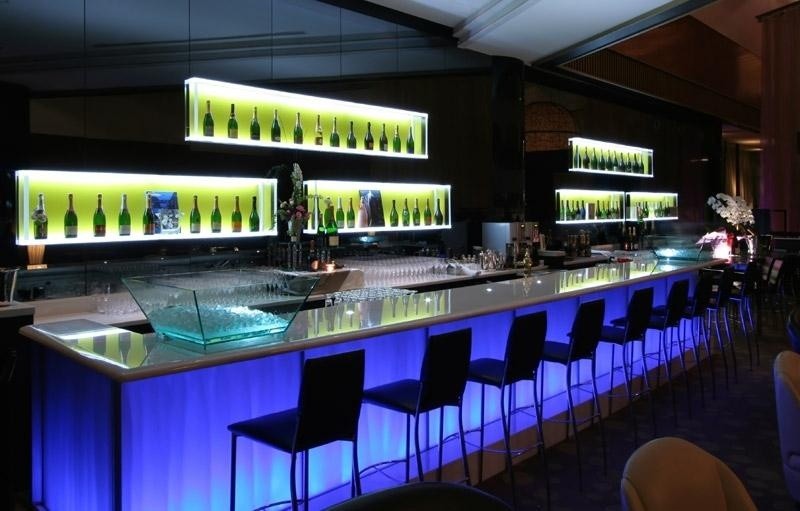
[
  {"x1": 275, "y1": 164, "x2": 312, "y2": 242},
  {"x1": 706, "y1": 189, "x2": 761, "y2": 239}
]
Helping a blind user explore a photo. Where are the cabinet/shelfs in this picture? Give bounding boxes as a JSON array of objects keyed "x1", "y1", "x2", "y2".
[
  {"x1": 552, "y1": 134, "x2": 682, "y2": 225},
  {"x1": 12, "y1": 75, "x2": 454, "y2": 246}
]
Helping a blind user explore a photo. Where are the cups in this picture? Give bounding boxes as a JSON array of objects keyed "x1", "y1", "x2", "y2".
[
  {"x1": 90, "y1": 272, "x2": 290, "y2": 318},
  {"x1": 337, "y1": 255, "x2": 450, "y2": 288},
  {"x1": 325, "y1": 264, "x2": 335, "y2": 272}
]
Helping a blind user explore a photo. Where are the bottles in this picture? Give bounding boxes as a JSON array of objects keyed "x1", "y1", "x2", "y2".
[
  {"x1": 480, "y1": 224, "x2": 543, "y2": 272},
  {"x1": 563, "y1": 146, "x2": 671, "y2": 258},
  {"x1": 196, "y1": 99, "x2": 418, "y2": 155},
  {"x1": 26, "y1": 189, "x2": 451, "y2": 270},
  {"x1": 300, "y1": 290, "x2": 458, "y2": 334},
  {"x1": 549, "y1": 260, "x2": 619, "y2": 288}
]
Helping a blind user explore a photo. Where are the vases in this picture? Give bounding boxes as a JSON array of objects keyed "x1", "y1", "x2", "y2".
[{"x1": 735, "y1": 233, "x2": 759, "y2": 260}]
